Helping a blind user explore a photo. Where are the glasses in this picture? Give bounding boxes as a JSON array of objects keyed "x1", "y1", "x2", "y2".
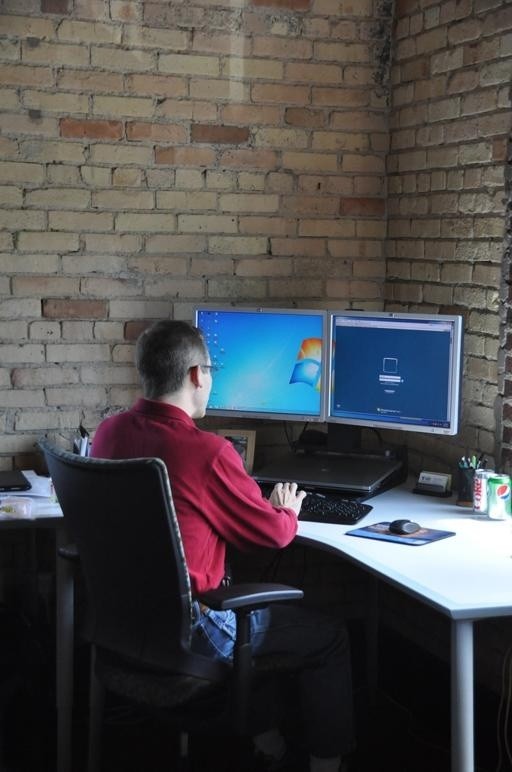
[{"x1": 187, "y1": 365, "x2": 218, "y2": 375}]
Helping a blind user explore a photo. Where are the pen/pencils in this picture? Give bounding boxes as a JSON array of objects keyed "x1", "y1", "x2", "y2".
[{"x1": 458, "y1": 452, "x2": 488, "y2": 480}]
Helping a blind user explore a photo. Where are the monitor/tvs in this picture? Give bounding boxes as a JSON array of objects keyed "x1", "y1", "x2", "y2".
[
  {"x1": 191, "y1": 303, "x2": 326, "y2": 424},
  {"x1": 292, "y1": 309, "x2": 463, "y2": 453}
]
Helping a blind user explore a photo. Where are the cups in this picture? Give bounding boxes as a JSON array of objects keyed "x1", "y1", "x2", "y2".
[{"x1": 456, "y1": 468, "x2": 476, "y2": 507}]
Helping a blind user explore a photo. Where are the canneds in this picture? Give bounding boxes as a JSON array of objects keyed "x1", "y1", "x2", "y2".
[
  {"x1": 486, "y1": 474, "x2": 511, "y2": 521},
  {"x1": 472, "y1": 468, "x2": 497, "y2": 515}
]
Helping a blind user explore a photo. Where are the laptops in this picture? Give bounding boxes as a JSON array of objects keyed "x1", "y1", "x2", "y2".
[
  {"x1": 1, "y1": 469, "x2": 32, "y2": 494},
  {"x1": 250, "y1": 452, "x2": 403, "y2": 493}
]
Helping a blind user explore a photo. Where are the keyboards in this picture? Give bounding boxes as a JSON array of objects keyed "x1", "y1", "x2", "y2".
[{"x1": 257, "y1": 483, "x2": 373, "y2": 524}]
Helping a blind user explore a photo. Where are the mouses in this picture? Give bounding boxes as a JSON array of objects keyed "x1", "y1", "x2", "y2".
[{"x1": 390, "y1": 520, "x2": 420, "y2": 534}]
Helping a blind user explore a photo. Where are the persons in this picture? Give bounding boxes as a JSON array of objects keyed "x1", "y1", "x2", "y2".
[{"x1": 92, "y1": 319, "x2": 355, "y2": 771}]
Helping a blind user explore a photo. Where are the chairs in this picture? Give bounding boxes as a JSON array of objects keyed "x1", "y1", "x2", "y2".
[{"x1": 34, "y1": 440, "x2": 326, "y2": 772}]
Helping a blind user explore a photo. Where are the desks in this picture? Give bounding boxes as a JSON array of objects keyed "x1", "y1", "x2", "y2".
[{"x1": 1, "y1": 473, "x2": 512, "y2": 771}]
